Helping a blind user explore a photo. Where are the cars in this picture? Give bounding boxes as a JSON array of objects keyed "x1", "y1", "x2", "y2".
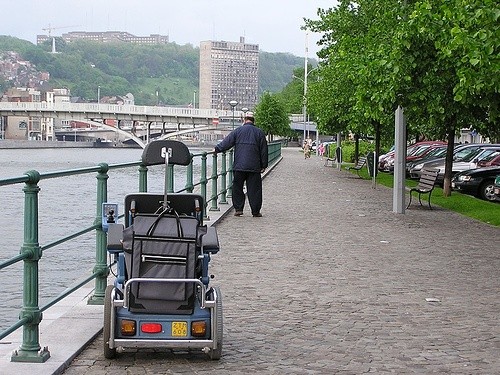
[
  {"x1": 378, "y1": 141, "x2": 500, "y2": 189},
  {"x1": 451, "y1": 165, "x2": 500, "y2": 204}
]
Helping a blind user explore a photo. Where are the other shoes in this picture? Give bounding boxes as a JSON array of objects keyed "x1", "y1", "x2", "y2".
[
  {"x1": 253, "y1": 213, "x2": 262, "y2": 217},
  {"x1": 234, "y1": 209, "x2": 243, "y2": 216}
]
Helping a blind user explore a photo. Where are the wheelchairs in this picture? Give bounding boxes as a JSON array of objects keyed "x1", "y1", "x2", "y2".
[{"x1": 103, "y1": 139, "x2": 225, "y2": 361}]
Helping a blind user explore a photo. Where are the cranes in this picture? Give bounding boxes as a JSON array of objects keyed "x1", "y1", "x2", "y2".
[{"x1": 42, "y1": 24, "x2": 82, "y2": 37}]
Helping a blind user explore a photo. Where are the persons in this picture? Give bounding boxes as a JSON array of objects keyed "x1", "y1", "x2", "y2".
[
  {"x1": 214, "y1": 116, "x2": 268, "y2": 216},
  {"x1": 319, "y1": 143, "x2": 325, "y2": 156},
  {"x1": 303, "y1": 141, "x2": 310, "y2": 159}
]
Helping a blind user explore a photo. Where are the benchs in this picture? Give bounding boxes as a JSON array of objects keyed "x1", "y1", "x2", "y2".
[
  {"x1": 345, "y1": 157, "x2": 367, "y2": 179},
  {"x1": 404, "y1": 167, "x2": 441, "y2": 210},
  {"x1": 324, "y1": 155, "x2": 336, "y2": 167}
]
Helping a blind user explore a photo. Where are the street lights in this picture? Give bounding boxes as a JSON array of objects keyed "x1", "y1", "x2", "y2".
[
  {"x1": 194, "y1": 90, "x2": 197, "y2": 109},
  {"x1": 229, "y1": 100, "x2": 238, "y2": 131},
  {"x1": 241, "y1": 107, "x2": 249, "y2": 123},
  {"x1": 98, "y1": 86, "x2": 101, "y2": 104},
  {"x1": 291, "y1": 65, "x2": 321, "y2": 140}
]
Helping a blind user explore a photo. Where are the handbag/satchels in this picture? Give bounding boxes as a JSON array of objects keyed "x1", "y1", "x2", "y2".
[{"x1": 129, "y1": 208, "x2": 197, "y2": 314}]
